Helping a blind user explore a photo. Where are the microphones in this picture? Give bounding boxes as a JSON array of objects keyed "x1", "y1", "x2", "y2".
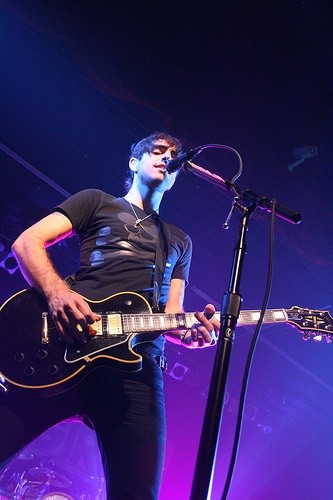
[{"x1": 166, "y1": 148, "x2": 205, "y2": 174}]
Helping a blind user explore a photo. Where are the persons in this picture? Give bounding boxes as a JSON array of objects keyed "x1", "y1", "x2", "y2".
[{"x1": 0, "y1": 131, "x2": 222, "y2": 500}]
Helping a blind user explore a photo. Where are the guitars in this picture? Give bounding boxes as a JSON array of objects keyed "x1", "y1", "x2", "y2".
[{"x1": 0, "y1": 285, "x2": 333, "y2": 398}]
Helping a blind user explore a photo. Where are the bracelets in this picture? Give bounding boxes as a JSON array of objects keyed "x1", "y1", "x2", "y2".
[{"x1": 180, "y1": 330, "x2": 188, "y2": 342}]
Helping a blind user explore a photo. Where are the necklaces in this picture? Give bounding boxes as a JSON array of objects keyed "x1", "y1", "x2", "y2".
[{"x1": 125, "y1": 195, "x2": 159, "y2": 231}]
